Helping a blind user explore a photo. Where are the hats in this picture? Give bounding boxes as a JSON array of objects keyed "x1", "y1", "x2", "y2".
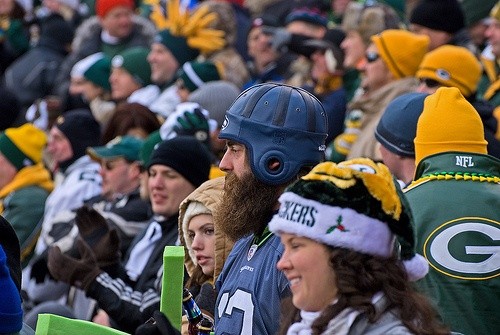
[
  {"x1": 190, "y1": 80, "x2": 240, "y2": 127},
  {"x1": 111, "y1": 46, "x2": 153, "y2": 88},
  {"x1": 266, "y1": 156, "x2": 416, "y2": 261},
  {"x1": 181, "y1": 200, "x2": 215, "y2": 266},
  {"x1": 54, "y1": 109, "x2": 104, "y2": 157},
  {"x1": 215, "y1": 82, "x2": 331, "y2": 182},
  {"x1": 372, "y1": 29, "x2": 432, "y2": 82},
  {"x1": 72, "y1": 52, "x2": 111, "y2": 93},
  {"x1": 339, "y1": 0, "x2": 402, "y2": 46},
  {"x1": 148, "y1": 134, "x2": 210, "y2": 185},
  {"x1": 0, "y1": 124, "x2": 51, "y2": 169},
  {"x1": 146, "y1": 0, "x2": 229, "y2": 68},
  {"x1": 95, "y1": 1, "x2": 131, "y2": 21},
  {"x1": 176, "y1": 61, "x2": 224, "y2": 92},
  {"x1": 413, "y1": 86, "x2": 489, "y2": 165},
  {"x1": 247, "y1": 8, "x2": 346, "y2": 65},
  {"x1": 416, "y1": 43, "x2": 485, "y2": 99},
  {"x1": 406, "y1": 0, "x2": 468, "y2": 31},
  {"x1": 83, "y1": 136, "x2": 145, "y2": 165},
  {"x1": 374, "y1": 92, "x2": 430, "y2": 158}
]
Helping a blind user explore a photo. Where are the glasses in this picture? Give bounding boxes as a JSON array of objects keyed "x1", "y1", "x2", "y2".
[{"x1": 96, "y1": 161, "x2": 133, "y2": 170}]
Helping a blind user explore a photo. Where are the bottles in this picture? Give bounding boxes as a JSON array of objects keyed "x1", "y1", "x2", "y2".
[{"x1": 182, "y1": 288, "x2": 215, "y2": 335}]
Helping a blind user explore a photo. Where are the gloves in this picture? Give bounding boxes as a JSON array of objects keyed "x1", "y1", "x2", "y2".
[
  {"x1": 72, "y1": 205, "x2": 122, "y2": 265},
  {"x1": 169, "y1": 108, "x2": 209, "y2": 141},
  {"x1": 44, "y1": 235, "x2": 103, "y2": 290},
  {"x1": 138, "y1": 313, "x2": 181, "y2": 335}
]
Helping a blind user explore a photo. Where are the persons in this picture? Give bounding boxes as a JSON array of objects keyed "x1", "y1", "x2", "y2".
[
  {"x1": 266, "y1": 157, "x2": 461, "y2": 335},
  {"x1": 0, "y1": 0, "x2": 500, "y2": 335}
]
